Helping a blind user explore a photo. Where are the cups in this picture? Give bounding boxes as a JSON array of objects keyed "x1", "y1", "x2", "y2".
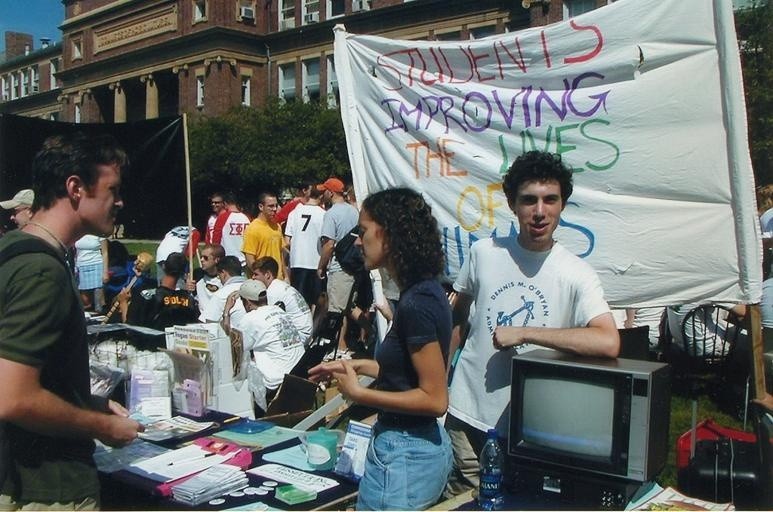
[{"x1": 307, "y1": 427, "x2": 337, "y2": 470}]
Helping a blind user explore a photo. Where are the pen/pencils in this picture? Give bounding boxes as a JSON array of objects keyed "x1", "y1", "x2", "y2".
[
  {"x1": 223, "y1": 416, "x2": 241, "y2": 423},
  {"x1": 168, "y1": 452, "x2": 216, "y2": 465}
]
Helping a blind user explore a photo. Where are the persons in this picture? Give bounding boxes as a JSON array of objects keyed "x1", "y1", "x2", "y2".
[
  {"x1": 612, "y1": 186, "x2": 773, "y2": 413},
  {"x1": 73, "y1": 177, "x2": 373, "y2": 416},
  {"x1": 0, "y1": 189, "x2": 35, "y2": 228},
  {"x1": 307, "y1": 186, "x2": 455, "y2": 512},
  {"x1": 443, "y1": 149, "x2": 620, "y2": 489},
  {"x1": 0, "y1": 131, "x2": 146, "y2": 512}
]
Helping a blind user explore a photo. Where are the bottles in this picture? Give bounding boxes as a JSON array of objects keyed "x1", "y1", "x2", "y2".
[{"x1": 479, "y1": 429, "x2": 505, "y2": 510}]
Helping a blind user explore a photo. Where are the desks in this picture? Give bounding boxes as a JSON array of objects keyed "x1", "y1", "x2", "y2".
[{"x1": 93, "y1": 410, "x2": 360, "y2": 510}]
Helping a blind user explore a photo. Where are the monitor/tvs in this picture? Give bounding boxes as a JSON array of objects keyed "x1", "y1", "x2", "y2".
[{"x1": 507, "y1": 350, "x2": 671, "y2": 482}]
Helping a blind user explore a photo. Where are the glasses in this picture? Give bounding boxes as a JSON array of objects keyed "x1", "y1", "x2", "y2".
[{"x1": 200, "y1": 255, "x2": 213, "y2": 261}]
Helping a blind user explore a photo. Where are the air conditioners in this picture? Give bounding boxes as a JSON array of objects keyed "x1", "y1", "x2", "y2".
[
  {"x1": 241, "y1": 6, "x2": 254, "y2": 18},
  {"x1": 304, "y1": 13, "x2": 319, "y2": 21}
]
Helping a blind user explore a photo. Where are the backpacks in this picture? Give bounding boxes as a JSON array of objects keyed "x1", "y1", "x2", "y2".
[{"x1": 336, "y1": 225, "x2": 364, "y2": 275}]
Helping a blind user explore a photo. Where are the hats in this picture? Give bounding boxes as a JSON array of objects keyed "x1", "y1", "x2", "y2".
[
  {"x1": 0, "y1": 189, "x2": 35, "y2": 209},
  {"x1": 239, "y1": 279, "x2": 267, "y2": 301},
  {"x1": 317, "y1": 178, "x2": 344, "y2": 193}
]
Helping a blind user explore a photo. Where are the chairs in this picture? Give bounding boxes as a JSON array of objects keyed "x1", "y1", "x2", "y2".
[
  {"x1": 743, "y1": 325, "x2": 773, "y2": 434},
  {"x1": 681, "y1": 304, "x2": 739, "y2": 411},
  {"x1": 269, "y1": 312, "x2": 343, "y2": 412}
]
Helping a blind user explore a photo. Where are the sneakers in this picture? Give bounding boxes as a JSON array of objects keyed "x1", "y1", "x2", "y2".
[{"x1": 322, "y1": 348, "x2": 352, "y2": 361}]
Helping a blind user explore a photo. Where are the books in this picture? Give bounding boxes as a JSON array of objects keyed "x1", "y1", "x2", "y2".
[{"x1": 629, "y1": 487, "x2": 736, "y2": 512}]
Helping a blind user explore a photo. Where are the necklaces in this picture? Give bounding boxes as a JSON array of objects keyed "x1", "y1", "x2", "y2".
[{"x1": 25, "y1": 219, "x2": 71, "y2": 259}]
